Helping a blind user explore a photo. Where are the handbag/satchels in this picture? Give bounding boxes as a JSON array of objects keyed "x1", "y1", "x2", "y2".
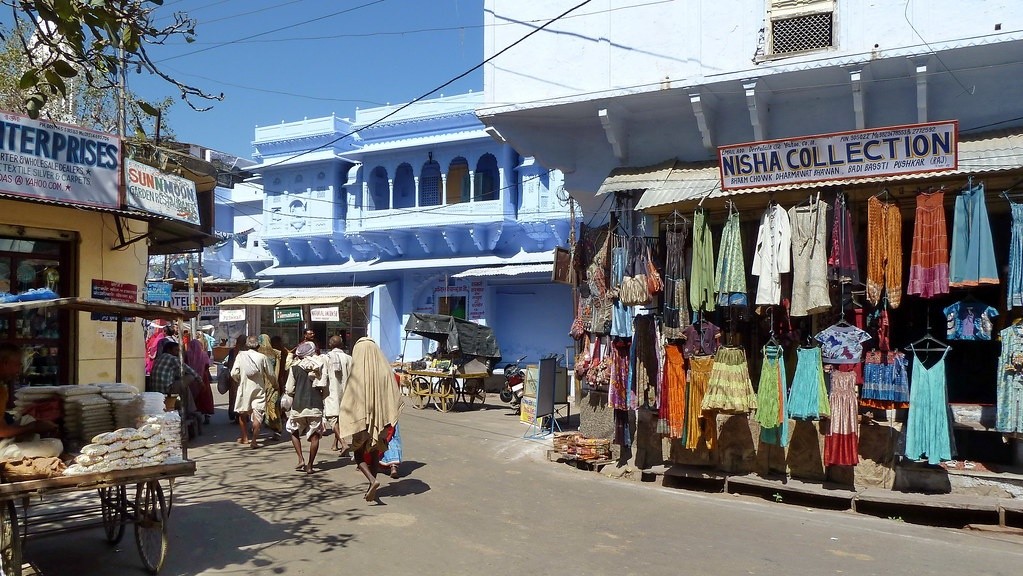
[
  {"x1": 573, "y1": 335, "x2": 611, "y2": 387},
  {"x1": 280, "y1": 393, "x2": 293, "y2": 411},
  {"x1": 568, "y1": 222, "x2": 611, "y2": 340},
  {"x1": 620, "y1": 247, "x2": 665, "y2": 306},
  {"x1": 218, "y1": 368, "x2": 229, "y2": 394}
]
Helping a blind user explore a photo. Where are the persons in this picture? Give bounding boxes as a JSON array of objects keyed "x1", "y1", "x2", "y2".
[
  {"x1": 231, "y1": 335, "x2": 278, "y2": 448},
  {"x1": 224, "y1": 334, "x2": 247, "y2": 424},
  {"x1": 258, "y1": 334, "x2": 290, "y2": 437},
  {"x1": 350, "y1": 422, "x2": 403, "y2": 478},
  {"x1": 152, "y1": 326, "x2": 216, "y2": 423},
  {"x1": 285, "y1": 342, "x2": 323, "y2": 473},
  {"x1": 0, "y1": 341, "x2": 58, "y2": 438},
  {"x1": 290, "y1": 328, "x2": 319, "y2": 359},
  {"x1": 339, "y1": 336, "x2": 405, "y2": 501},
  {"x1": 312, "y1": 336, "x2": 352, "y2": 456}
]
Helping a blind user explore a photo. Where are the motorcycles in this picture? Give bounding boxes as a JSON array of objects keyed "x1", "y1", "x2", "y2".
[{"x1": 500, "y1": 355, "x2": 527, "y2": 416}]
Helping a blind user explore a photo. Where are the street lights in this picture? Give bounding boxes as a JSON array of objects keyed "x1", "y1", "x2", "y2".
[{"x1": 136, "y1": 100, "x2": 161, "y2": 146}]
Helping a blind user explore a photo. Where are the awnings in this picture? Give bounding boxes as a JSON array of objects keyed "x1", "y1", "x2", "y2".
[
  {"x1": 451, "y1": 264, "x2": 554, "y2": 278},
  {"x1": 594, "y1": 128, "x2": 1022, "y2": 211},
  {"x1": 220, "y1": 286, "x2": 373, "y2": 305},
  {"x1": 0, "y1": 189, "x2": 223, "y2": 255}
]
